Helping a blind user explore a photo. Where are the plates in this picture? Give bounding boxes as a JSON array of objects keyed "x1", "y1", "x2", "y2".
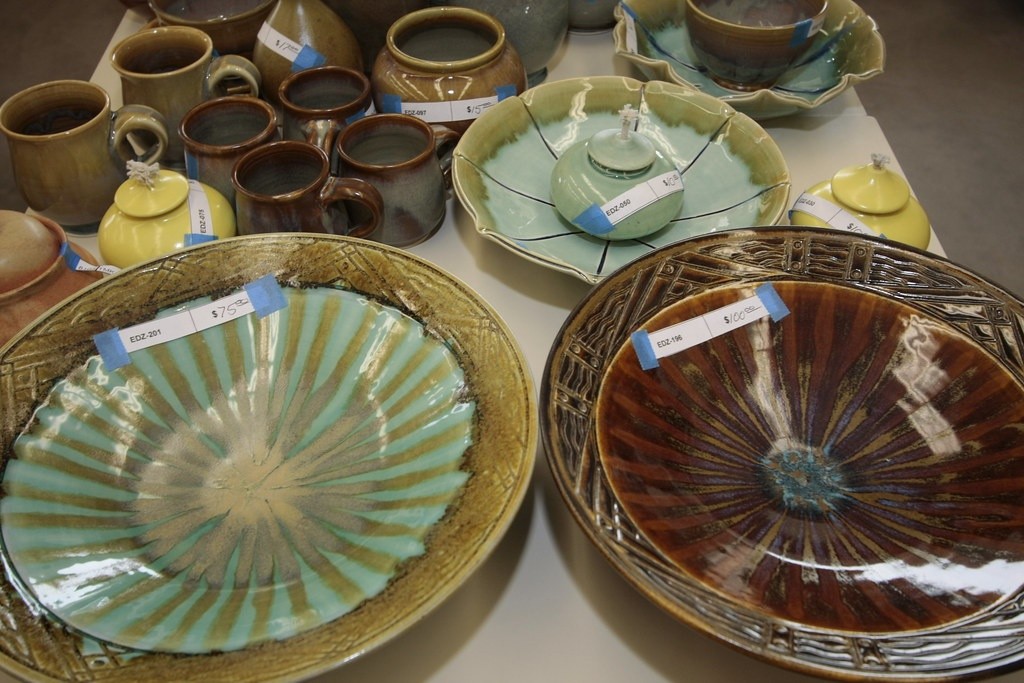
[
  {"x1": 609, "y1": 0, "x2": 886, "y2": 119},
  {"x1": 451, "y1": 74, "x2": 794, "y2": 287},
  {"x1": 0, "y1": 229, "x2": 536, "y2": 683},
  {"x1": 537, "y1": 225, "x2": 1024, "y2": 683}
]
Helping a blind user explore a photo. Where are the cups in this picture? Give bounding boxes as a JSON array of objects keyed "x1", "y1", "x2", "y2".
[
  {"x1": 229, "y1": 139, "x2": 385, "y2": 239},
  {"x1": 137, "y1": 135, "x2": 152, "y2": 137},
  {"x1": 275, "y1": 65, "x2": 378, "y2": 160},
  {"x1": 0, "y1": 78, "x2": 171, "y2": 227},
  {"x1": 336, "y1": 112, "x2": 462, "y2": 250},
  {"x1": 683, "y1": 0, "x2": 829, "y2": 94},
  {"x1": 176, "y1": 95, "x2": 281, "y2": 211},
  {"x1": 109, "y1": 24, "x2": 263, "y2": 161}
]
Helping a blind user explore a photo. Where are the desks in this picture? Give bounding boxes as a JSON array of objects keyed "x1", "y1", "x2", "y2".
[{"x1": 0, "y1": 1, "x2": 1024, "y2": 682}]
